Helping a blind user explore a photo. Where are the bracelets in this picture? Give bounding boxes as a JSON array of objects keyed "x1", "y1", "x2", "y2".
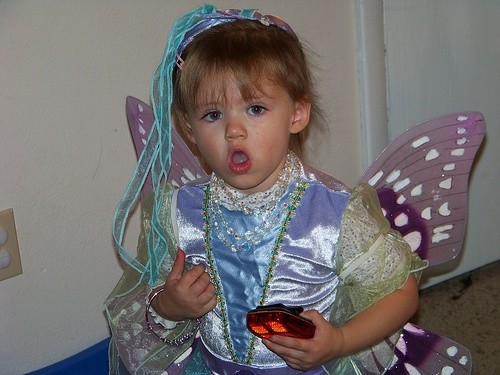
[{"x1": 145, "y1": 288, "x2": 202, "y2": 347}]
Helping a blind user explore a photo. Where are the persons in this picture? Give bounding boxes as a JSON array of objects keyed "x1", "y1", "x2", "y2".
[{"x1": 100, "y1": 4, "x2": 489, "y2": 374}]
